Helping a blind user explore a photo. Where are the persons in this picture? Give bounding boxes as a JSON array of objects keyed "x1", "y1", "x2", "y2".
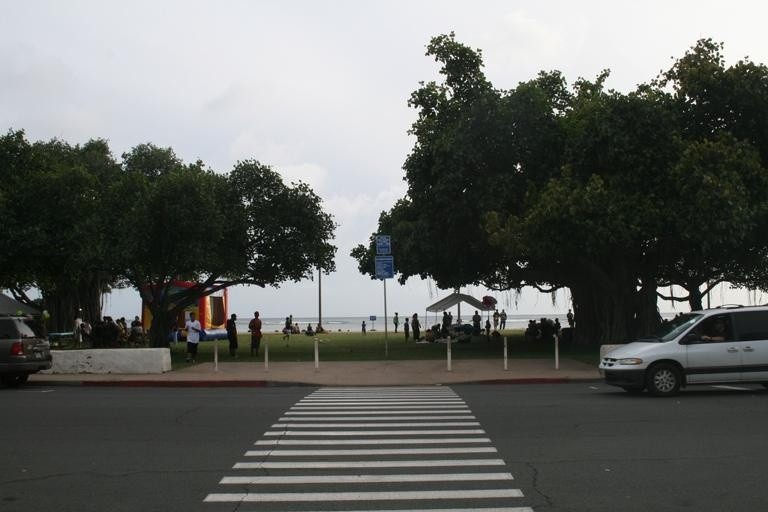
[
  {"x1": 171, "y1": 320, "x2": 178, "y2": 345},
  {"x1": 412, "y1": 313, "x2": 420, "y2": 340},
  {"x1": 289, "y1": 314, "x2": 294, "y2": 334},
  {"x1": 411, "y1": 314, "x2": 421, "y2": 337},
  {"x1": 392, "y1": 312, "x2": 399, "y2": 333},
  {"x1": 70, "y1": 311, "x2": 151, "y2": 350},
  {"x1": 499, "y1": 309, "x2": 506, "y2": 330},
  {"x1": 484, "y1": 320, "x2": 491, "y2": 336},
  {"x1": 523, "y1": 317, "x2": 560, "y2": 342},
  {"x1": 315, "y1": 323, "x2": 323, "y2": 333},
  {"x1": 403, "y1": 317, "x2": 409, "y2": 343},
  {"x1": 493, "y1": 309, "x2": 499, "y2": 329},
  {"x1": 247, "y1": 310, "x2": 262, "y2": 357},
  {"x1": 360, "y1": 320, "x2": 366, "y2": 335},
  {"x1": 471, "y1": 310, "x2": 480, "y2": 327},
  {"x1": 566, "y1": 308, "x2": 574, "y2": 329},
  {"x1": 307, "y1": 323, "x2": 312, "y2": 330},
  {"x1": 293, "y1": 323, "x2": 299, "y2": 334},
  {"x1": 183, "y1": 313, "x2": 202, "y2": 363},
  {"x1": 282, "y1": 316, "x2": 290, "y2": 348},
  {"x1": 699, "y1": 318, "x2": 729, "y2": 342},
  {"x1": 422, "y1": 311, "x2": 459, "y2": 343},
  {"x1": 226, "y1": 313, "x2": 239, "y2": 358}
]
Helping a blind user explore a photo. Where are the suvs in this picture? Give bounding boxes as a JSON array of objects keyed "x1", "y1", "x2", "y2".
[{"x1": 0, "y1": 311, "x2": 53, "y2": 389}]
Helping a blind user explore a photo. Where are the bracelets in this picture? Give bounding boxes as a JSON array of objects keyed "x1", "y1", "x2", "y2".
[{"x1": 710, "y1": 336, "x2": 713, "y2": 340}]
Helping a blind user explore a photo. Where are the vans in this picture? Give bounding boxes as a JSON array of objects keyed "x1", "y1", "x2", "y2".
[{"x1": 598, "y1": 305, "x2": 768, "y2": 397}]
